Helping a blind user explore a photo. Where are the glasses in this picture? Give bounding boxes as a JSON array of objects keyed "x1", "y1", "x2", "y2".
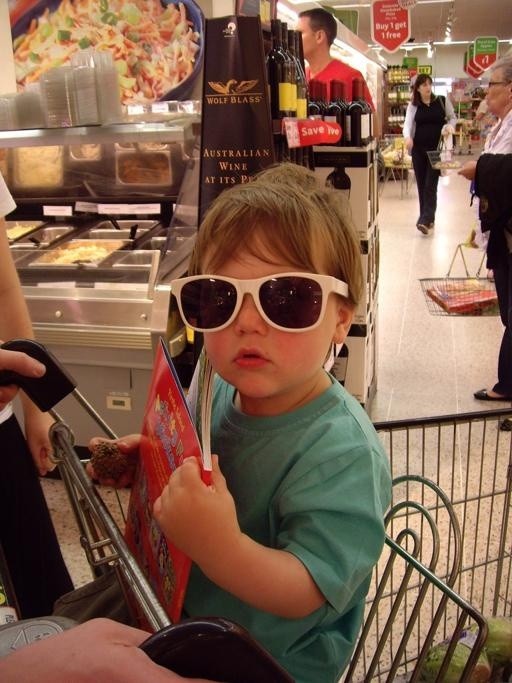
[
  {"x1": 169, "y1": 269, "x2": 351, "y2": 333},
  {"x1": 487, "y1": 80, "x2": 506, "y2": 87}
]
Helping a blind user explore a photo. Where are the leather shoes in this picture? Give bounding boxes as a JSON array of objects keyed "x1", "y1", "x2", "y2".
[
  {"x1": 500, "y1": 417, "x2": 511, "y2": 430},
  {"x1": 473, "y1": 387, "x2": 510, "y2": 400}
]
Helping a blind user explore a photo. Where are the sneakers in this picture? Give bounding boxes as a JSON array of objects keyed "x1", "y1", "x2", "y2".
[
  {"x1": 429, "y1": 220, "x2": 434, "y2": 229},
  {"x1": 416, "y1": 221, "x2": 429, "y2": 233}
]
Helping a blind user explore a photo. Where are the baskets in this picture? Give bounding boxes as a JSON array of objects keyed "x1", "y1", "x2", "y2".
[
  {"x1": 426, "y1": 130, "x2": 471, "y2": 170},
  {"x1": 417, "y1": 242, "x2": 501, "y2": 318}
]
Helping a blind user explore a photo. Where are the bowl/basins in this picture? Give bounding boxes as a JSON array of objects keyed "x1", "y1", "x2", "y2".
[{"x1": 11, "y1": 0, "x2": 206, "y2": 102}]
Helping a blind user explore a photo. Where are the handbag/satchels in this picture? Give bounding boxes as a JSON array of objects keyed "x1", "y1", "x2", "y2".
[{"x1": 438, "y1": 96, "x2": 457, "y2": 147}]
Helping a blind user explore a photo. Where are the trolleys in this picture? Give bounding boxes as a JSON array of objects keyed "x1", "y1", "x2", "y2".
[
  {"x1": 380, "y1": 134, "x2": 413, "y2": 199},
  {"x1": 1, "y1": 341, "x2": 512, "y2": 683}
]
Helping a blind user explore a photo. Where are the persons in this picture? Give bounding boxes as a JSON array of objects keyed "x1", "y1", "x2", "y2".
[
  {"x1": 0, "y1": 618, "x2": 214, "y2": 682},
  {"x1": 1, "y1": 168, "x2": 76, "y2": 623},
  {"x1": 457, "y1": 52, "x2": 512, "y2": 433},
  {"x1": 83, "y1": 163, "x2": 391, "y2": 683},
  {"x1": 295, "y1": 9, "x2": 378, "y2": 140},
  {"x1": 403, "y1": 75, "x2": 456, "y2": 236},
  {"x1": 474, "y1": 87, "x2": 497, "y2": 123}
]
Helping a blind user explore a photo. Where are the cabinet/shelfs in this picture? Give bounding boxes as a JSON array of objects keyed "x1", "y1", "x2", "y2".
[
  {"x1": 0, "y1": 104, "x2": 205, "y2": 449},
  {"x1": 383, "y1": 65, "x2": 416, "y2": 180}
]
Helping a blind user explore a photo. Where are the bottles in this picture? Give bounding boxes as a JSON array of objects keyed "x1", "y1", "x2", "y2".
[
  {"x1": 384, "y1": 65, "x2": 409, "y2": 82},
  {"x1": 266, "y1": 18, "x2": 308, "y2": 120},
  {"x1": 325, "y1": 166, "x2": 350, "y2": 199},
  {"x1": 307, "y1": 77, "x2": 374, "y2": 146}
]
